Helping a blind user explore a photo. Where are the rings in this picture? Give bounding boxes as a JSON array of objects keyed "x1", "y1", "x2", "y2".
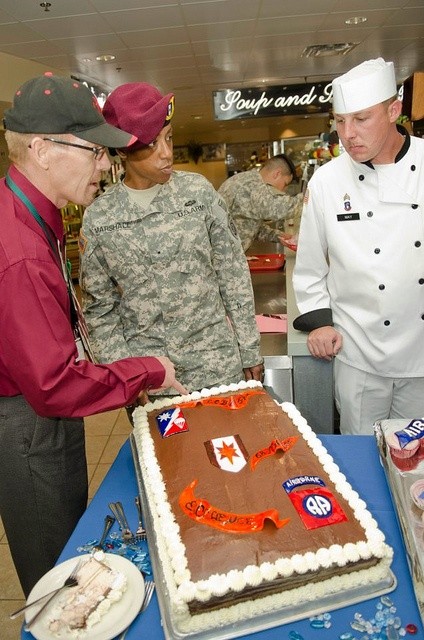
[{"x1": 262, "y1": 372, "x2": 266, "y2": 377}]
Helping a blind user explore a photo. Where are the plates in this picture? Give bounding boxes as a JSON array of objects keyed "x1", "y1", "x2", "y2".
[
  {"x1": 128, "y1": 429, "x2": 398, "y2": 640},
  {"x1": 25, "y1": 553, "x2": 145, "y2": 640},
  {"x1": 243, "y1": 254, "x2": 287, "y2": 270},
  {"x1": 279, "y1": 236, "x2": 297, "y2": 252}
]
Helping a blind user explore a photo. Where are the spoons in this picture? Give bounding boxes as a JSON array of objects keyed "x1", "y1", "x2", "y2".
[{"x1": 90, "y1": 514, "x2": 115, "y2": 562}]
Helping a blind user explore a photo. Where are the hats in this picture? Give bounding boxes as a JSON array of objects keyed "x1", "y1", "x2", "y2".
[
  {"x1": 2, "y1": 72, "x2": 138, "y2": 149},
  {"x1": 102, "y1": 82, "x2": 176, "y2": 156},
  {"x1": 332, "y1": 57, "x2": 398, "y2": 115}
]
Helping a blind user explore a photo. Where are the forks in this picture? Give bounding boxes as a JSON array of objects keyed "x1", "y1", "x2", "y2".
[
  {"x1": 109, "y1": 502, "x2": 135, "y2": 545},
  {"x1": 118, "y1": 581, "x2": 155, "y2": 640},
  {"x1": 135, "y1": 496, "x2": 146, "y2": 541},
  {"x1": 9, "y1": 558, "x2": 84, "y2": 619}
]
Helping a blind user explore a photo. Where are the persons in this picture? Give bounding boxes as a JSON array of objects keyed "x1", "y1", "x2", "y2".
[
  {"x1": 79, "y1": 83, "x2": 265, "y2": 427},
  {"x1": 216, "y1": 153, "x2": 303, "y2": 254},
  {"x1": 291, "y1": 58, "x2": 424, "y2": 436},
  {"x1": 0, "y1": 71, "x2": 189, "y2": 602}
]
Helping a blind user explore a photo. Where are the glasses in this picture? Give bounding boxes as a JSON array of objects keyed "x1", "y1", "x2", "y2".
[{"x1": 28, "y1": 138, "x2": 107, "y2": 161}]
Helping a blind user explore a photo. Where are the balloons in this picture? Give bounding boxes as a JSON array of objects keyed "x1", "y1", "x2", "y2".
[
  {"x1": 332, "y1": 142, "x2": 339, "y2": 156},
  {"x1": 308, "y1": 147, "x2": 327, "y2": 159},
  {"x1": 329, "y1": 144, "x2": 336, "y2": 156}
]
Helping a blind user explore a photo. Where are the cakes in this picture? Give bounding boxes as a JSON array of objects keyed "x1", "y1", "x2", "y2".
[
  {"x1": 47, "y1": 565, "x2": 129, "y2": 639},
  {"x1": 131, "y1": 379, "x2": 396, "y2": 636},
  {"x1": 375, "y1": 417, "x2": 424, "y2": 617}
]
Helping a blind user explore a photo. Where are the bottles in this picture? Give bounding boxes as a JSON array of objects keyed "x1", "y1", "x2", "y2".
[{"x1": 288, "y1": 218, "x2": 294, "y2": 227}]
